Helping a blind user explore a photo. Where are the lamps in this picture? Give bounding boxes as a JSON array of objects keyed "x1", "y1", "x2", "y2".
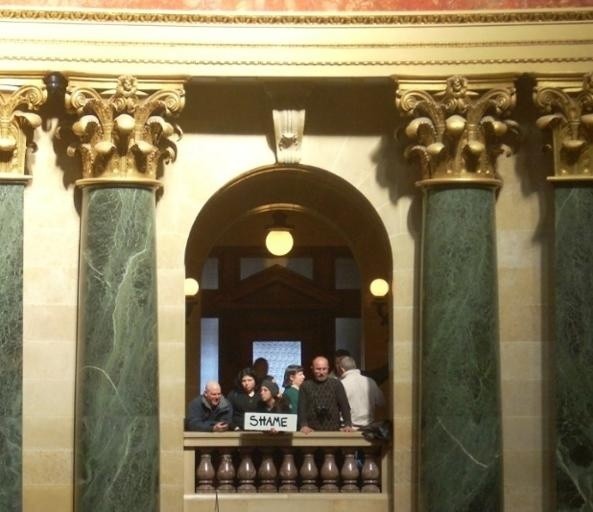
[
  {"x1": 185, "y1": 276, "x2": 200, "y2": 319},
  {"x1": 262, "y1": 209, "x2": 297, "y2": 256},
  {"x1": 365, "y1": 276, "x2": 391, "y2": 325}
]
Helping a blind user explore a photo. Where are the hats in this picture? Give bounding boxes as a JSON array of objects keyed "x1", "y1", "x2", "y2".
[{"x1": 261, "y1": 380, "x2": 278, "y2": 397}]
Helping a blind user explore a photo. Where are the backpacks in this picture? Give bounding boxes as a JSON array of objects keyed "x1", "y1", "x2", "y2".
[{"x1": 358, "y1": 419, "x2": 392, "y2": 458}]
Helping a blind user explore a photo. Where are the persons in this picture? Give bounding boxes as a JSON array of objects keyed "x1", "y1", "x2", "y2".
[
  {"x1": 296, "y1": 356, "x2": 355, "y2": 433},
  {"x1": 258, "y1": 376, "x2": 293, "y2": 433},
  {"x1": 281, "y1": 365, "x2": 307, "y2": 431},
  {"x1": 337, "y1": 353, "x2": 387, "y2": 487},
  {"x1": 248, "y1": 358, "x2": 274, "y2": 394},
  {"x1": 225, "y1": 366, "x2": 264, "y2": 431},
  {"x1": 184, "y1": 380, "x2": 234, "y2": 434},
  {"x1": 326, "y1": 348, "x2": 350, "y2": 381}
]
